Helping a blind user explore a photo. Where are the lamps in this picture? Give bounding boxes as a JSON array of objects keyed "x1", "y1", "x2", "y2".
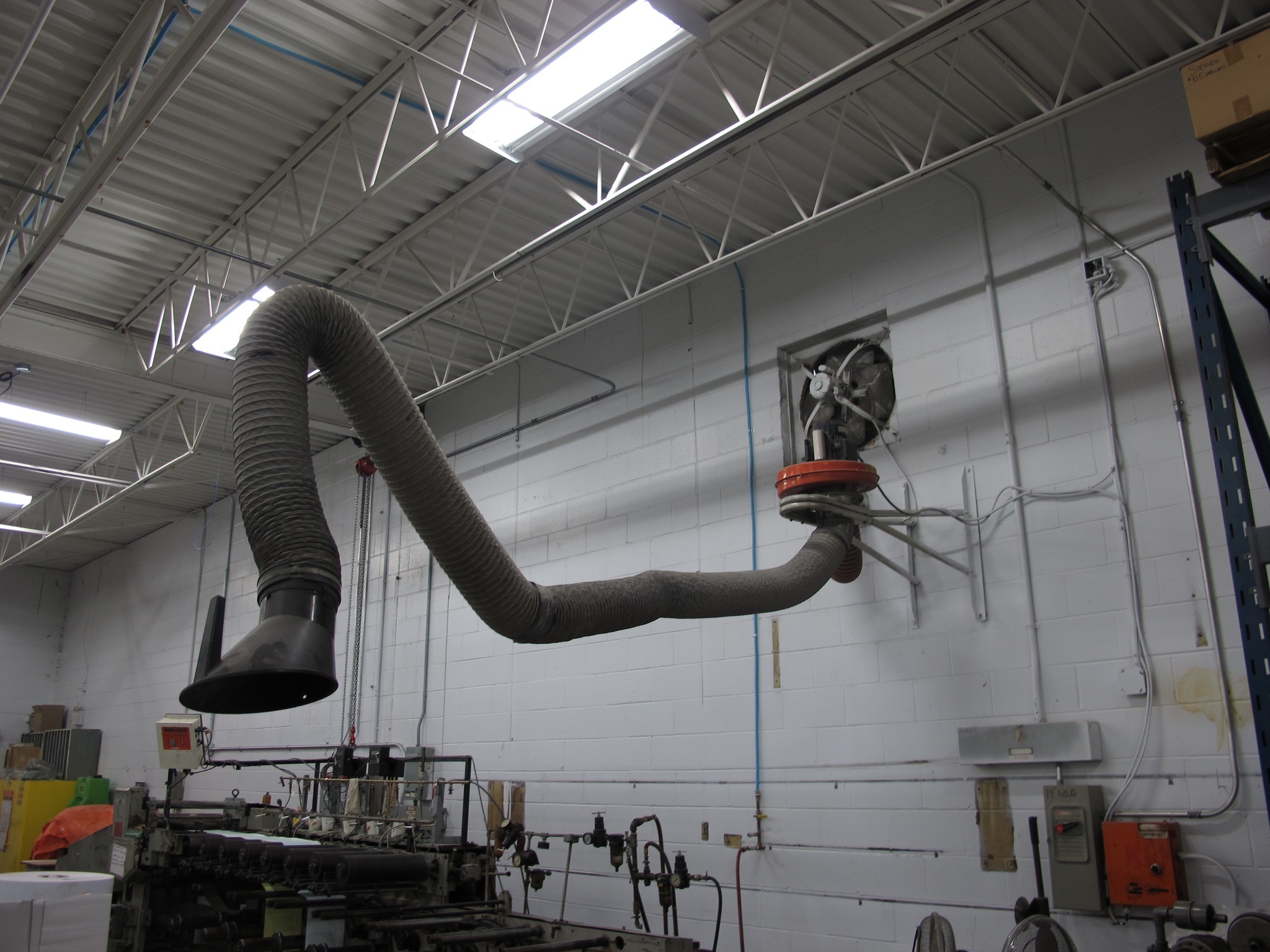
[
  {"x1": 0, "y1": 468, "x2": 33, "y2": 512},
  {"x1": 0, "y1": 371, "x2": 123, "y2": 446},
  {"x1": 192, "y1": 280, "x2": 293, "y2": 361},
  {"x1": 457, "y1": 0, "x2": 716, "y2": 165}
]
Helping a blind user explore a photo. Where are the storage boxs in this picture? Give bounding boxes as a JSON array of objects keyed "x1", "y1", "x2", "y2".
[
  {"x1": 32, "y1": 703, "x2": 68, "y2": 733},
  {"x1": 2, "y1": 741, "x2": 42, "y2": 770}
]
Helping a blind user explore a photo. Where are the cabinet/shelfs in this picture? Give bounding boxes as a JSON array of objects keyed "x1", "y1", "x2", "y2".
[{"x1": 20, "y1": 725, "x2": 101, "y2": 780}]
